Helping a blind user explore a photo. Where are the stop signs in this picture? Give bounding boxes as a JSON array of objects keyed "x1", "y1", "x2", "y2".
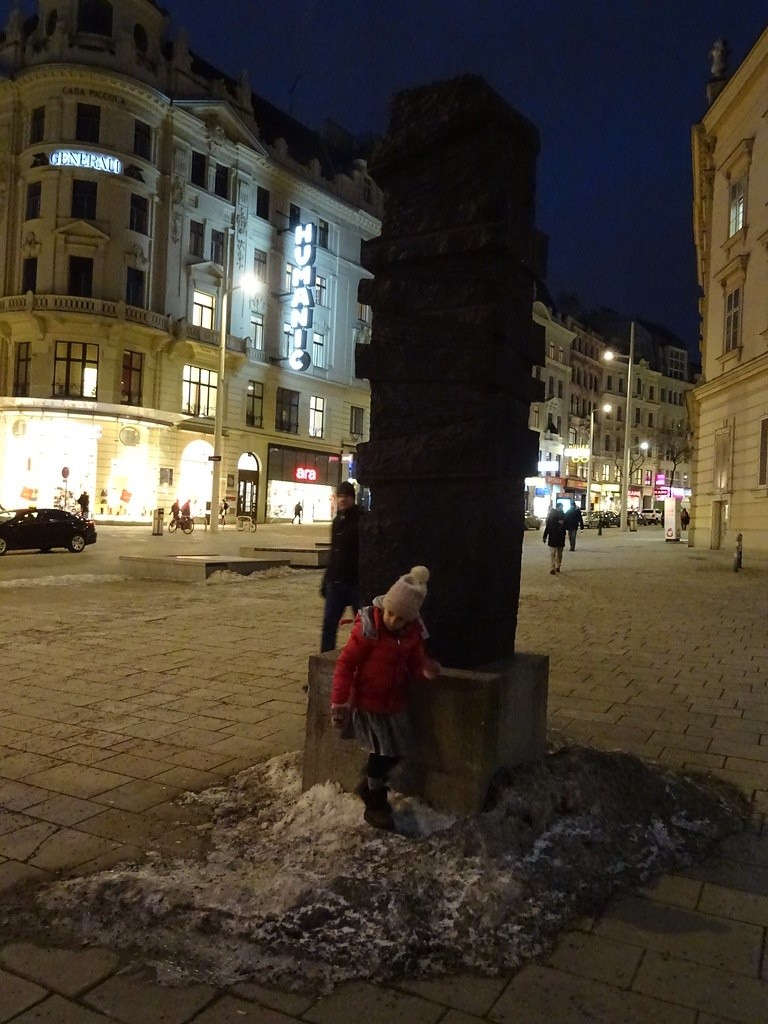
[{"x1": 61, "y1": 468, "x2": 69, "y2": 478}]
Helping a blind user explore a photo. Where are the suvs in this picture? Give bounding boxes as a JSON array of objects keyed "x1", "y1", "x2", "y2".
[{"x1": 619, "y1": 509, "x2": 662, "y2": 526}]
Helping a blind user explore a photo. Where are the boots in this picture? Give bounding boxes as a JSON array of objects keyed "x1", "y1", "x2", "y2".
[{"x1": 355, "y1": 780, "x2": 396, "y2": 830}]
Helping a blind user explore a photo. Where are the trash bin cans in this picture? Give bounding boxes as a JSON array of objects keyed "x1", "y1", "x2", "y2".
[{"x1": 152, "y1": 508, "x2": 165, "y2": 536}]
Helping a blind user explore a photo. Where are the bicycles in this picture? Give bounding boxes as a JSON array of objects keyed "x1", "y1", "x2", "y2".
[
  {"x1": 168, "y1": 513, "x2": 194, "y2": 534},
  {"x1": 237, "y1": 519, "x2": 257, "y2": 533}
]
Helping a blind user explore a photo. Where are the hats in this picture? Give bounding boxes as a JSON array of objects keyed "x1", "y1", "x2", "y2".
[
  {"x1": 336, "y1": 481, "x2": 356, "y2": 495},
  {"x1": 383, "y1": 565, "x2": 430, "y2": 619}
]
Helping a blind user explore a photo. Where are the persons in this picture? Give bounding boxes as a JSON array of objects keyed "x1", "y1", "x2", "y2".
[
  {"x1": 169, "y1": 498, "x2": 181, "y2": 518},
  {"x1": 302, "y1": 481, "x2": 371, "y2": 693},
  {"x1": 680, "y1": 508, "x2": 688, "y2": 530},
  {"x1": 179, "y1": 500, "x2": 191, "y2": 516},
  {"x1": 543, "y1": 503, "x2": 569, "y2": 574},
  {"x1": 77, "y1": 491, "x2": 89, "y2": 518},
  {"x1": 330, "y1": 566, "x2": 443, "y2": 830},
  {"x1": 565, "y1": 501, "x2": 584, "y2": 551},
  {"x1": 292, "y1": 501, "x2": 303, "y2": 525},
  {"x1": 219, "y1": 500, "x2": 228, "y2": 525}
]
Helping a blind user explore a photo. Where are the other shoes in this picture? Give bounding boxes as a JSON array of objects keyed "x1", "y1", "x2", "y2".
[
  {"x1": 556, "y1": 568, "x2": 560, "y2": 572},
  {"x1": 550, "y1": 569, "x2": 555, "y2": 575}
]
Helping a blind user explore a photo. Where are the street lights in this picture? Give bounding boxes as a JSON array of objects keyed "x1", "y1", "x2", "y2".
[
  {"x1": 605, "y1": 351, "x2": 632, "y2": 531},
  {"x1": 206, "y1": 226, "x2": 262, "y2": 533},
  {"x1": 626, "y1": 442, "x2": 649, "y2": 505},
  {"x1": 586, "y1": 406, "x2": 613, "y2": 510}
]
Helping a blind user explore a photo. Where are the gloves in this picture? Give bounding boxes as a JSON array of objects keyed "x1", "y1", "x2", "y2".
[{"x1": 331, "y1": 707, "x2": 350, "y2": 729}]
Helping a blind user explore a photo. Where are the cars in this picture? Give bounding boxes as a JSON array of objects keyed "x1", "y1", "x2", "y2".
[
  {"x1": 525, "y1": 511, "x2": 541, "y2": 529},
  {"x1": 0, "y1": 508, "x2": 97, "y2": 555},
  {"x1": 579, "y1": 510, "x2": 619, "y2": 528}
]
